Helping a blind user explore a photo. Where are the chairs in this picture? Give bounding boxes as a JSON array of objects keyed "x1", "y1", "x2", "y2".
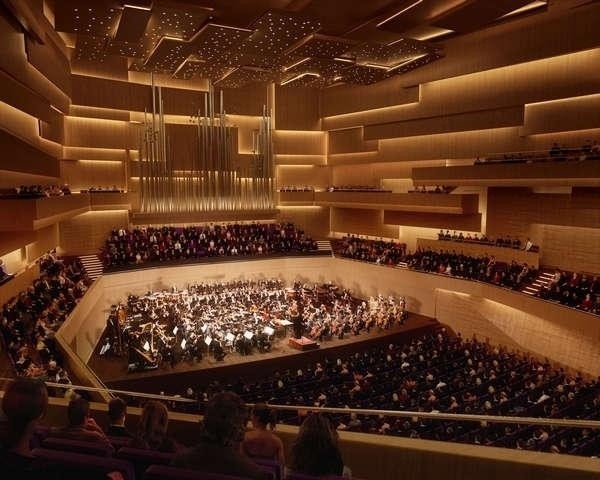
[{"x1": 1, "y1": 429, "x2": 284, "y2": 479}]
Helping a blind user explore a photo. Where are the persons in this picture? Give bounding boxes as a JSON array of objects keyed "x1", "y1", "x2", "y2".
[
  {"x1": 472, "y1": 139, "x2": 600, "y2": 164},
  {"x1": 278, "y1": 183, "x2": 455, "y2": 193},
  {"x1": 1, "y1": 222, "x2": 599, "y2": 478},
  {"x1": 1, "y1": 178, "x2": 123, "y2": 202}
]
